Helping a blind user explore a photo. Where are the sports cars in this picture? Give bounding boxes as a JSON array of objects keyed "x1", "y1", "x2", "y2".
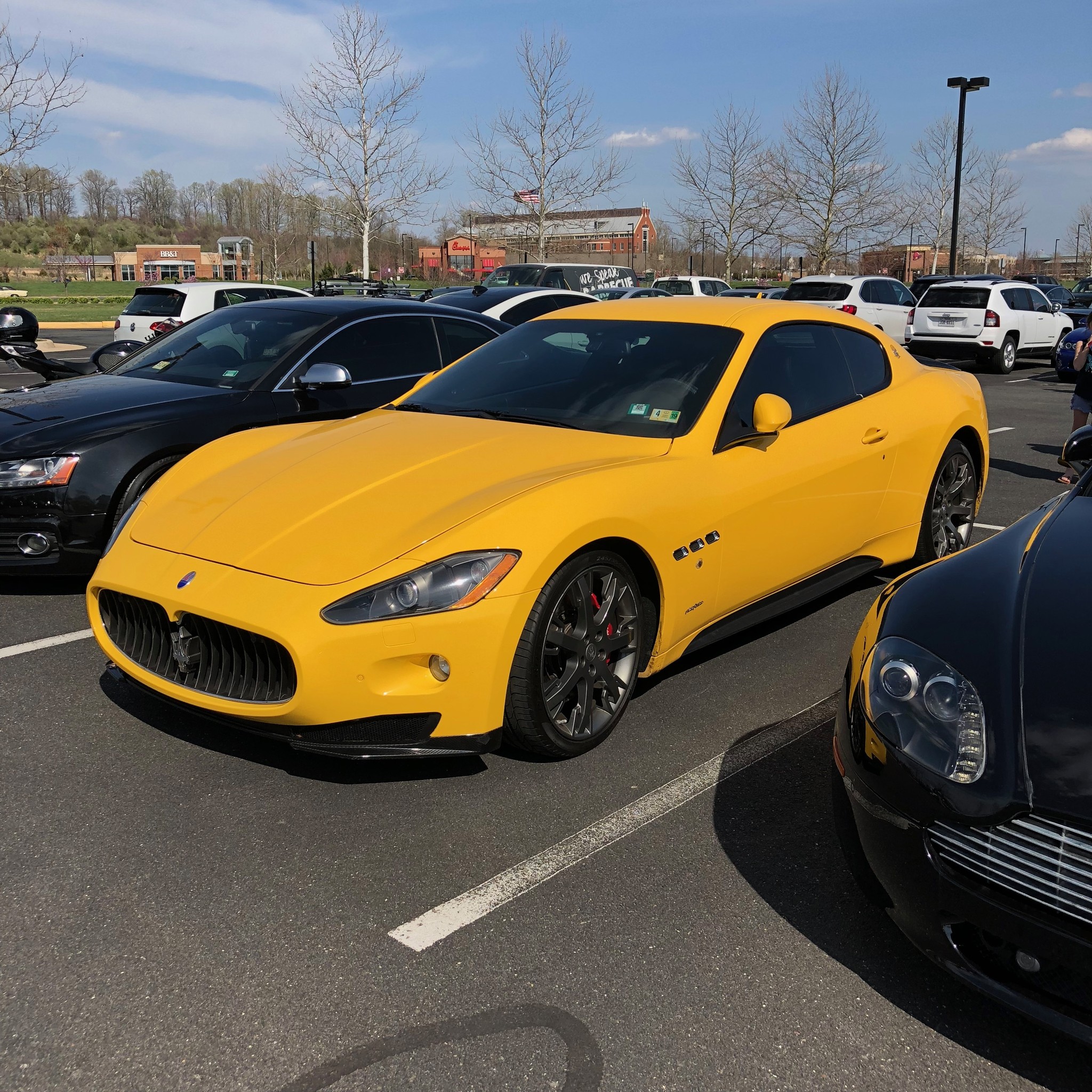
[{"x1": 83, "y1": 296, "x2": 992, "y2": 764}]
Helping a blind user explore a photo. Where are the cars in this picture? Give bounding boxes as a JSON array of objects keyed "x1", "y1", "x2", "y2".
[
  {"x1": 0, "y1": 286, "x2": 28, "y2": 298},
  {"x1": 912, "y1": 273, "x2": 1092, "y2": 380},
  {"x1": 830, "y1": 426, "x2": 1091, "y2": 1040},
  {"x1": 53, "y1": 278, "x2": 71, "y2": 283},
  {"x1": 418, "y1": 262, "x2": 783, "y2": 353},
  {"x1": 0, "y1": 294, "x2": 577, "y2": 575},
  {"x1": 113, "y1": 282, "x2": 320, "y2": 357}
]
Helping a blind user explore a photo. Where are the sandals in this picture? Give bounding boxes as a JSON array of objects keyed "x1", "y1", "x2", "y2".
[{"x1": 1058, "y1": 474, "x2": 1071, "y2": 484}]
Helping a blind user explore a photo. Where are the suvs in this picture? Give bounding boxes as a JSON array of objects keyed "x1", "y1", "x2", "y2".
[
  {"x1": 905, "y1": 280, "x2": 1074, "y2": 374},
  {"x1": 300, "y1": 284, "x2": 344, "y2": 296},
  {"x1": 780, "y1": 275, "x2": 918, "y2": 346}
]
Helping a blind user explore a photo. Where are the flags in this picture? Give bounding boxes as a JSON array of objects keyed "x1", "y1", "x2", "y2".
[{"x1": 514, "y1": 186, "x2": 541, "y2": 203}]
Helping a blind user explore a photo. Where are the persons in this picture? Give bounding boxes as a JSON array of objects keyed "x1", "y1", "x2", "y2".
[{"x1": 1058, "y1": 311, "x2": 1092, "y2": 484}]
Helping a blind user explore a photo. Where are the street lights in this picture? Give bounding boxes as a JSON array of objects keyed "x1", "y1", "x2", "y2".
[
  {"x1": 777, "y1": 235, "x2": 783, "y2": 279},
  {"x1": 671, "y1": 237, "x2": 677, "y2": 271},
  {"x1": 962, "y1": 236, "x2": 968, "y2": 271},
  {"x1": 907, "y1": 224, "x2": 913, "y2": 282},
  {"x1": 1054, "y1": 239, "x2": 1061, "y2": 272},
  {"x1": 467, "y1": 214, "x2": 473, "y2": 280},
  {"x1": 90, "y1": 230, "x2": 96, "y2": 282},
  {"x1": 408, "y1": 237, "x2": 413, "y2": 272},
  {"x1": 713, "y1": 232, "x2": 720, "y2": 278},
  {"x1": 1074, "y1": 224, "x2": 1085, "y2": 278},
  {"x1": 402, "y1": 234, "x2": 407, "y2": 281},
  {"x1": 612, "y1": 223, "x2": 634, "y2": 270},
  {"x1": 1020, "y1": 228, "x2": 1027, "y2": 272},
  {"x1": 327, "y1": 235, "x2": 332, "y2": 267},
  {"x1": 918, "y1": 235, "x2": 924, "y2": 245},
  {"x1": 857, "y1": 241, "x2": 861, "y2": 276},
  {"x1": 946, "y1": 76, "x2": 990, "y2": 276}
]
